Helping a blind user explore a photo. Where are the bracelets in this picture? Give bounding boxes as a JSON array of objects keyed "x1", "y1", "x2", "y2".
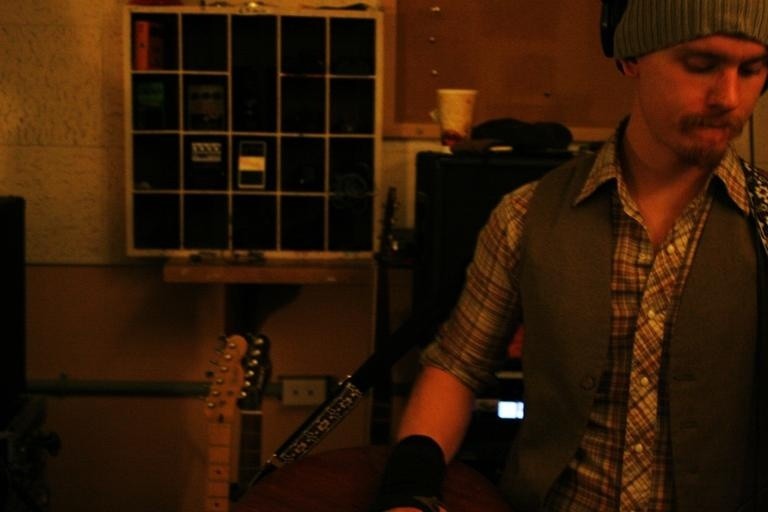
[{"x1": 374, "y1": 434, "x2": 448, "y2": 511}]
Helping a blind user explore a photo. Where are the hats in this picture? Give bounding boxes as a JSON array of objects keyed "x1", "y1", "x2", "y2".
[{"x1": 613, "y1": 1, "x2": 768, "y2": 60}]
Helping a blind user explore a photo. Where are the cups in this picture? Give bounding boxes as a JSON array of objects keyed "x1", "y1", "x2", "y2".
[{"x1": 436, "y1": 86, "x2": 479, "y2": 151}]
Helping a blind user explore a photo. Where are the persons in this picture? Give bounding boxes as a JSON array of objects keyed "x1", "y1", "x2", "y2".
[{"x1": 366, "y1": 2, "x2": 768, "y2": 510}]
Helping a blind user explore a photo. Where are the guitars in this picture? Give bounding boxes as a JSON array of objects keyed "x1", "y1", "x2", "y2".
[{"x1": 179, "y1": 330, "x2": 273, "y2": 512}]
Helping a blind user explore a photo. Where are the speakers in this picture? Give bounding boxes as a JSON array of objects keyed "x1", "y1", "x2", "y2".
[
  {"x1": 0, "y1": 196, "x2": 26, "y2": 433},
  {"x1": 413, "y1": 151, "x2": 575, "y2": 351}
]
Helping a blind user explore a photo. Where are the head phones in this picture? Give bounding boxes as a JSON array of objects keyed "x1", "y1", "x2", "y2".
[{"x1": 599, "y1": 0, "x2": 627, "y2": 59}]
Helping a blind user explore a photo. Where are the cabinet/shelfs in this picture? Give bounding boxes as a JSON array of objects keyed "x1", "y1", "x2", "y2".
[
  {"x1": 387, "y1": 138, "x2": 600, "y2": 452},
  {"x1": 120, "y1": 3, "x2": 397, "y2": 268}
]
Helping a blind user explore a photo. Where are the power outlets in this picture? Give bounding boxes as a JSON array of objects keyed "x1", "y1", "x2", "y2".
[{"x1": 280, "y1": 378, "x2": 328, "y2": 407}]
